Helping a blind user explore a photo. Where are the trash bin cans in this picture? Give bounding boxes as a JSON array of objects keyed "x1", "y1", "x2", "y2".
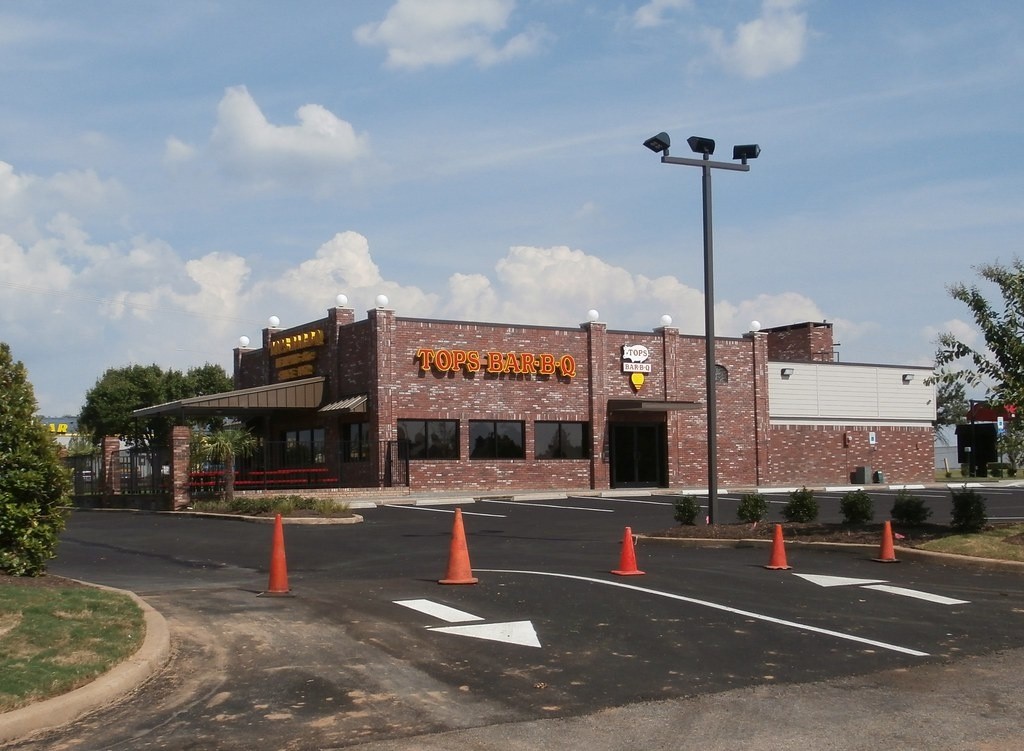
[
  {"x1": 875, "y1": 471, "x2": 883, "y2": 483},
  {"x1": 856, "y1": 466, "x2": 872, "y2": 484}
]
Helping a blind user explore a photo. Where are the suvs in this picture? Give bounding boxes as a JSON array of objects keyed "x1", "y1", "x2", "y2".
[{"x1": 72, "y1": 470, "x2": 96, "y2": 483}]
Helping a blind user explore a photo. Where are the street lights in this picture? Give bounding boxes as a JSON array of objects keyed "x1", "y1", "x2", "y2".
[{"x1": 643, "y1": 132, "x2": 760, "y2": 525}]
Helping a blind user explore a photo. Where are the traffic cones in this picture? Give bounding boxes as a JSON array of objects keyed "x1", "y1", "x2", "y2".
[
  {"x1": 871, "y1": 520, "x2": 901, "y2": 562},
  {"x1": 762, "y1": 524, "x2": 793, "y2": 570},
  {"x1": 256, "y1": 514, "x2": 297, "y2": 597},
  {"x1": 438, "y1": 507, "x2": 478, "y2": 584},
  {"x1": 609, "y1": 527, "x2": 645, "y2": 575}
]
